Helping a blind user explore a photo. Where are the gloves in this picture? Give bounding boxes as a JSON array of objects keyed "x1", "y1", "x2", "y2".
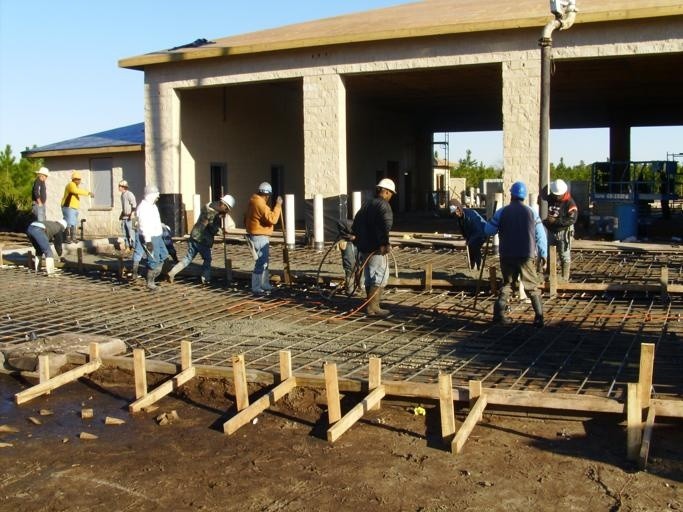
[
  {"x1": 539, "y1": 256, "x2": 548, "y2": 273},
  {"x1": 543, "y1": 216, "x2": 555, "y2": 225}
]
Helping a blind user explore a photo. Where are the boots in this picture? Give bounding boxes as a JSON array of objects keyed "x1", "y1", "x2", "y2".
[
  {"x1": 493, "y1": 298, "x2": 512, "y2": 324},
  {"x1": 560, "y1": 262, "x2": 570, "y2": 282},
  {"x1": 131, "y1": 262, "x2": 163, "y2": 290},
  {"x1": 65, "y1": 226, "x2": 80, "y2": 244},
  {"x1": 346, "y1": 269, "x2": 390, "y2": 317},
  {"x1": 34, "y1": 256, "x2": 44, "y2": 272},
  {"x1": 45, "y1": 257, "x2": 58, "y2": 277},
  {"x1": 531, "y1": 294, "x2": 544, "y2": 328}
]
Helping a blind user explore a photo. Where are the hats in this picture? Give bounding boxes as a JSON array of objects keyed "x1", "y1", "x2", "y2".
[{"x1": 447, "y1": 199, "x2": 463, "y2": 218}]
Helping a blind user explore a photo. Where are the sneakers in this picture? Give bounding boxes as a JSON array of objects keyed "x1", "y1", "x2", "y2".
[
  {"x1": 256, "y1": 287, "x2": 279, "y2": 296},
  {"x1": 201, "y1": 275, "x2": 208, "y2": 285},
  {"x1": 168, "y1": 272, "x2": 175, "y2": 283}
]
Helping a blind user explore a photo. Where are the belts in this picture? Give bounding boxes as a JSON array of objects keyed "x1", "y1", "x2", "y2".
[{"x1": 380, "y1": 244, "x2": 392, "y2": 255}]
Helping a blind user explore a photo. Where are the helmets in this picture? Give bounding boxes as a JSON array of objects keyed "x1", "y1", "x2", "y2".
[
  {"x1": 550, "y1": 178, "x2": 568, "y2": 195},
  {"x1": 220, "y1": 194, "x2": 236, "y2": 209},
  {"x1": 57, "y1": 219, "x2": 68, "y2": 229},
  {"x1": 377, "y1": 178, "x2": 397, "y2": 194},
  {"x1": 119, "y1": 179, "x2": 130, "y2": 188},
  {"x1": 145, "y1": 185, "x2": 159, "y2": 196},
  {"x1": 510, "y1": 182, "x2": 527, "y2": 199},
  {"x1": 35, "y1": 167, "x2": 49, "y2": 177},
  {"x1": 258, "y1": 182, "x2": 273, "y2": 195},
  {"x1": 72, "y1": 172, "x2": 81, "y2": 180}
]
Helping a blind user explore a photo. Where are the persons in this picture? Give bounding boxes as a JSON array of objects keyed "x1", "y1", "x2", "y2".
[
  {"x1": 244, "y1": 181, "x2": 285, "y2": 296},
  {"x1": 537, "y1": 178, "x2": 577, "y2": 280},
  {"x1": 659, "y1": 171, "x2": 674, "y2": 219},
  {"x1": 131, "y1": 221, "x2": 179, "y2": 281},
  {"x1": 165, "y1": 194, "x2": 235, "y2": 285},
  {"x1": 336, "y1": 218, "x2": 366, "y2": 297},
  {"x1": 352, "y1": 177, "x2": 397, "y2": 317},
  {"x1": 634, "y1": 172, "x2": 653, "y2": 205},
  {"x1": 118, "y1": 180, "x2": 137, "y2": 254},
  {"x1": 27, "y1": 218, "x2": 67, "y2": 278},
  {"x1": 135, "y1": 185, "x2": 168, "y2": 291},
  {"x1": 447, "y1": 198, "x2": 488, "y2": 271},
  {"x1": 60, "y1": 171, "x2": 97, "y2": 243},
  {"x1": 483, "y1": 180, "x2": 548, "y2": 329},
  {"x1": 31, "y1": 169, "x2": 50, "y2": 222}
]
